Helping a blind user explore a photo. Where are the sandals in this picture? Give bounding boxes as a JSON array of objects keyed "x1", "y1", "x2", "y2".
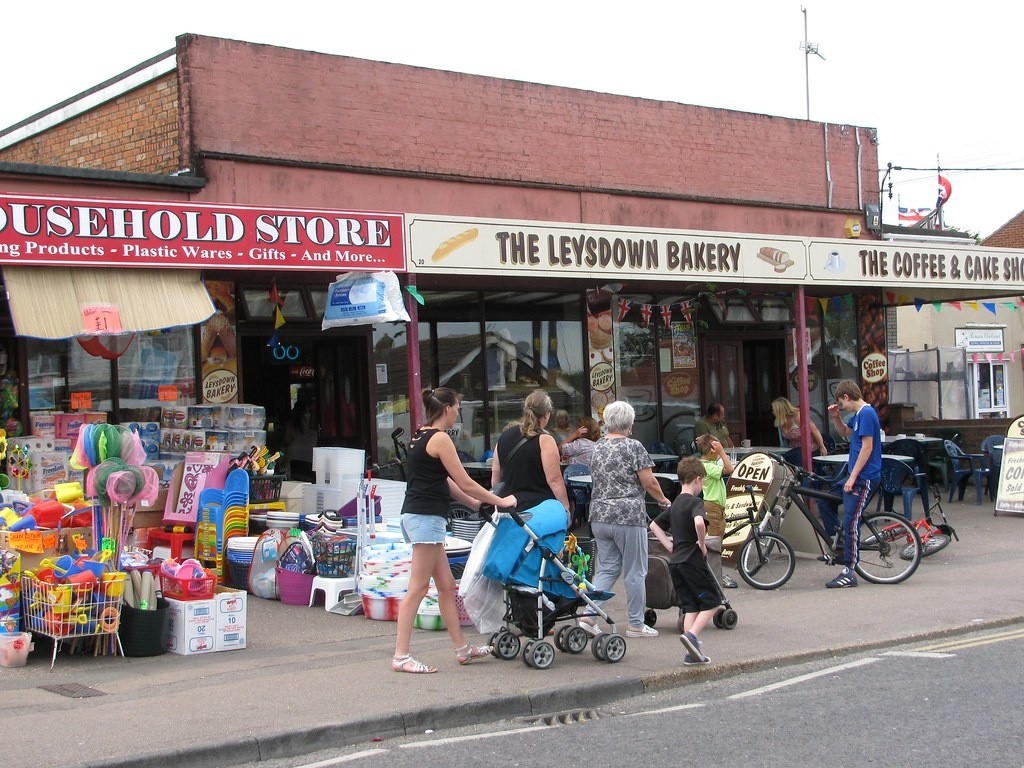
[
  {"x1": 393, "y1": 653, "x2": 438, "y2": 673},
  {"x1": 454, "y1": 643, "x2": 494, "y2": 665}
]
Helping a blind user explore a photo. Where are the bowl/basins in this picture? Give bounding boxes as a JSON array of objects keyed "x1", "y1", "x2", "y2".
[
  {"x1": 161, "y1": 557, "x2": 208, "y2": 594},
  {"x1": 361, "y1": 543, "x2": 411, "y2": 620},
  {"x1": 413, "y1": 610, "x2": 447, "y2": 630}
]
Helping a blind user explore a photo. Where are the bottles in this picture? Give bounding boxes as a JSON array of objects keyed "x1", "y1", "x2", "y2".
[{"x1": 164, "y1": 526, "x2": 192, "y2": 534}]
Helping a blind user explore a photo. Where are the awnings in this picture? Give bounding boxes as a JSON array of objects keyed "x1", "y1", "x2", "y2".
[{"x1": 0, "y1": 195, "x2": 1024, "y2": 292}]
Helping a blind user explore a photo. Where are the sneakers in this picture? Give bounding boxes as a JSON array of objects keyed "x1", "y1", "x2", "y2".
[
  {"x1": 684, "y1": 655, "x2": 710, "y2": 666},
  {"x1": 816, "y1": 554, "x2": 842, "y2": 561},
  {"x1": 680, "y1": 631, "x2": 704, "y2": 662},
  {"x1": 826, "y1": 567, "x2": 858, "y2": 588}
]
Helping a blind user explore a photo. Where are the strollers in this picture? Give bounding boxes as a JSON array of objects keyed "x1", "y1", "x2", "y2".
[{"x1": 479, "y1": 494, "x2": 627, "y2": 671}]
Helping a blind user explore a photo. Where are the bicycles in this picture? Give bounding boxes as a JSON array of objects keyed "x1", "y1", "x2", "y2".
[
  {"x1": 862, "y1": 491, "x2": 960, "y2": 558},
  {"x1": 649, "y1": 477, "x2": 796, "y2": 591},
  {"x1": 750, "y1": 452, "x2": 923, "y2": 585}
]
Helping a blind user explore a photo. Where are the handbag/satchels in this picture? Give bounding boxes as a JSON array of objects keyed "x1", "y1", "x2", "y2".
[
  {"x1": 457, "y1": 513, "x2": 506, "y2": 635},
  {"x1": 321, "y1": 271, "x2": 411, "y2": 332}
]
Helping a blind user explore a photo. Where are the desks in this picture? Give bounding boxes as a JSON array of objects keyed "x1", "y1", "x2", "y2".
[
  {"x1": 881, "y1": 435, "x2": 943, "y2": 451},
  {"x1": 648, "y1": 451, "x2": 679, "y2": 463},
  {"x1": 993, "y1": 444, "x2": 1003, "y2": 449},
  {"x1": 459, "y1": 461, "x2": 492, "y2": 470},
  {"x1": 812, "y1": 454, "x2": 914, "y2": 464},
  {"x1": 567, "y1": 473, "x2": 678, "y2": 483},
  {"x1": 725, "y1": 446, "x2": 792, "y2": 454}
]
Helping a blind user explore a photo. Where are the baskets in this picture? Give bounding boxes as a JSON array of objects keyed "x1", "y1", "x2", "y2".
[
  {"x1": 21, "y1": 575, "x2": 125, "y2": 638},
  {"x1": 249, "y1": 475, "x2": 284, "y2": 503},
  {"x1": 226, "y1": 537, "x2": 259, "y2": 594},
  {"x1": 455, "y1": 579, "x2": 474, "y2": 626},
  {"x1": 158, "y1": 572, "x2": 217, "y2": 601},
  {"x1": 309, "y1": 534, "x2": 357, "y2": 578},
  {"x1": 123, "y1": 565, "x2": 160, "y2": 580}
]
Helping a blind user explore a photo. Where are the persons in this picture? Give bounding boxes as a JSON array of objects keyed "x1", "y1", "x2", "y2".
[
  {"x1": 816, "y1": 380, "x2": 882, "y2": 588},
  {"x1": 391, "y1": 387, "x2": 520, "y2": 674},
  {"x1": 695, "y1": 434, "x2": 739, "y2": 589},
  {"x1": 491, "y1": 389, "x2": 573, "y2": 635},
  {"x1": 695, "y1": 404, "x2": 736, "y2": 450},
  {"x1": 282, "y1": 390, "x2": 318, "y2": 482},
  {"x1": 772, "y1": 397, "x2": 828, "y2": 466},
  {"x1": 580, "y1": 401, "x2": 671, "y2": 638},
  {"x1": 550, "y1": 408, "x2": 599, "y2": 503},
  {"x1": 649, "y1": 458, "x2": 721, "y2": 665}
]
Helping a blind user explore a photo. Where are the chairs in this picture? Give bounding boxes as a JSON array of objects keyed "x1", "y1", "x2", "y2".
[
  {"x1": 564, "y1": 484, "x2": 593, "y2": 537},
  {"x1": 193, "y1": 468, "x2": 250, "y2": 584},
  {"x1": 813, "y1": 428, "x2": 1004, "y2": 525},
  {"x1": 457, "y1": 441, "x2": 692, "y2": 535}
]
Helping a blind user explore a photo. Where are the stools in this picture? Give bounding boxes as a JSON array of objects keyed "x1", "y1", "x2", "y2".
[
  {"x1": 146, "y1": 528, "x2": 194, "y2": 565},
  {"x1": 250, "y1": 502, "x2": 287, "y2": 512},
  {"x1": 308, "y1": 574, "x2": 355, "y2": 612}
]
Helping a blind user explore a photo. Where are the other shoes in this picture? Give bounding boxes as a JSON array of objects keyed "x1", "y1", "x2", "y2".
[
  {"x1": 567, "y1": 517, "x2": 579, "y2": 532},
  {"x1": 721, "y1": 573, "x2": 737, "y2": 588},
  {"x1": 547, "y1": 629, "x2": 554, "y2": 635}
]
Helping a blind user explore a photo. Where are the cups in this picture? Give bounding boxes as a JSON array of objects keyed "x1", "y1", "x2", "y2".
[
  {"x1": 897, "y1": 434, "x2": 906, "y2": 439},
  {"x1": 915, "y1": 433, "x2": 925, "y2": 439},
  {"x1": 741, "y1": 440, "x2": 751, "y2": 448}
]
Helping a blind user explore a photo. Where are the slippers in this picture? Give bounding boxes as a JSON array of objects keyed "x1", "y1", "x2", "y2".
[
  {"x1": 625, "y1": 624, "x2": 659, "y2": 638},
  {"x1": 579, "y1": 621, "x2": 602, "y2": 636}
]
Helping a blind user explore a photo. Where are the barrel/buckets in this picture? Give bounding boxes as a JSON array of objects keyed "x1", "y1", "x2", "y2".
[
  {"x1": 117, "y1": 590, "x2": 171, "y2": 656},
  {"x1": 104, "y1": 572, "x2": 127, "y2": 596},
  {"x1": 6, "y1": 499, "x2": 75, "y2": 531},
  {"x1": 49, "y1": 590, "x2": 70, "y2": 613},
  {"x1": 275, "y1": 562, "x2": 315, "y2": 606},
  {"x1": 53, "y1": 555, "x2": 106, "y2": 582},
  {"x1": 249, "y1": 511, "x2": 357, "y2": 535},
  {"x1": 37, "y1": 569, "x2": 55, "y2": 585},
  {"x1": 562, "y1": 534, "x2": 595, "y2": 606},
  {"x1": 68, "y1": 569, "x2": 98, "y2": 596}
]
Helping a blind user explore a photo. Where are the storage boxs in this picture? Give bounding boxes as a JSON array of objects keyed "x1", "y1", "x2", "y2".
[
  {"x1": 250, "y1": 446, "x2": 407, "y2": 528},
  {"x1": 133, "y1": 450, "x2": 230, "y2": 529},
  {"x1": 123, "y1": 566, "x2": 247, "y2": 655},
  {"x1": 0, "y1": 526, "x2": 92, "y2": 667}
]
